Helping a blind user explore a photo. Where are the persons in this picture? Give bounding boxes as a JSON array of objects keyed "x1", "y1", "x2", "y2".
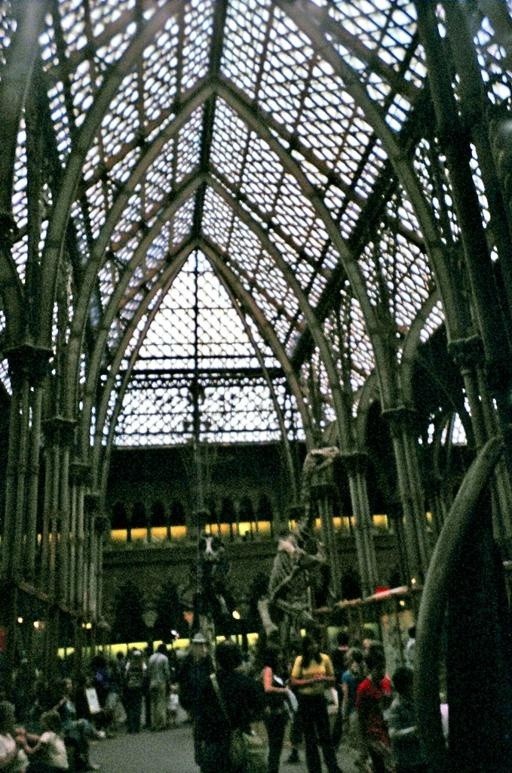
[
  {"x1": 94, "y1": 643, "x2": 182, "y2": 738},
  {"x1": 180, "y1": 625, "x2": 447, "y2": 773},
  {"x1": 1, "y1": 647, "x2": 107, "y2": 772}
]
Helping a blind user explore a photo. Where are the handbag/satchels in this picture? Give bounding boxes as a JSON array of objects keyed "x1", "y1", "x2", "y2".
[{"x1": 232, "y1": 728, "x2": 267, "y2": 772}]
